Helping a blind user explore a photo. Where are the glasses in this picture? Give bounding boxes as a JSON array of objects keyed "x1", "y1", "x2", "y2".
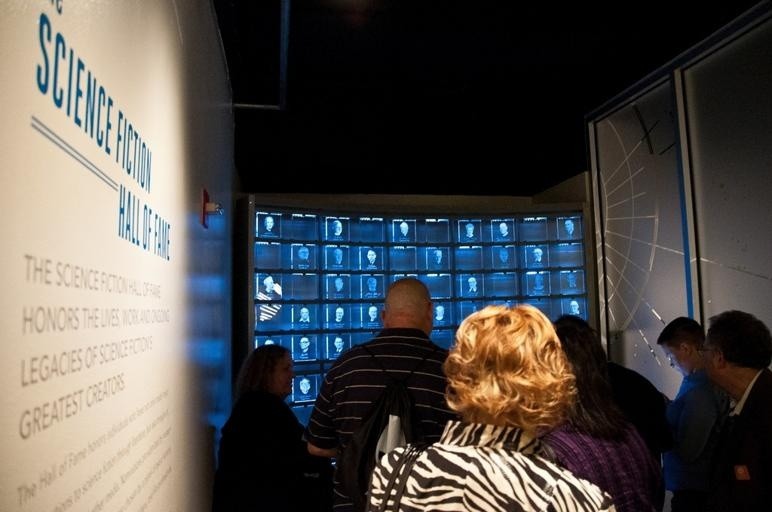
[{"x1": 697, "y1": 345, "x2": 716, "y2": 357}]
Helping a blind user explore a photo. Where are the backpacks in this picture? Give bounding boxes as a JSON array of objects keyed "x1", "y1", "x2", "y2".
[{"x1": 338, "y1": 344, "x2": 437, "y2": 512}]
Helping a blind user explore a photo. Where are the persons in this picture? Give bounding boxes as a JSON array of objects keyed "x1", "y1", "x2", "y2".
[
  {"x1": 554, "y1": 310, "x2": 675, "y2": 468},
  {"x1": 655, "y1": 318, "x2": 728, "y2": 494},
  {"x1": 533, "y1": 314, "x2": 663, "y2": 510},
  {"x1": 373, "y1": 302, "x2": 618, "y2": 511},
  {"x1": 673, "y1": 314, "x2": 770, "y2": 511},
  {"x1": 218, "y1": 343, "x2": 333, "y2": 511},
  {"x1": 305, "y1": 275, "x2": 460, "y2": 511}
]
